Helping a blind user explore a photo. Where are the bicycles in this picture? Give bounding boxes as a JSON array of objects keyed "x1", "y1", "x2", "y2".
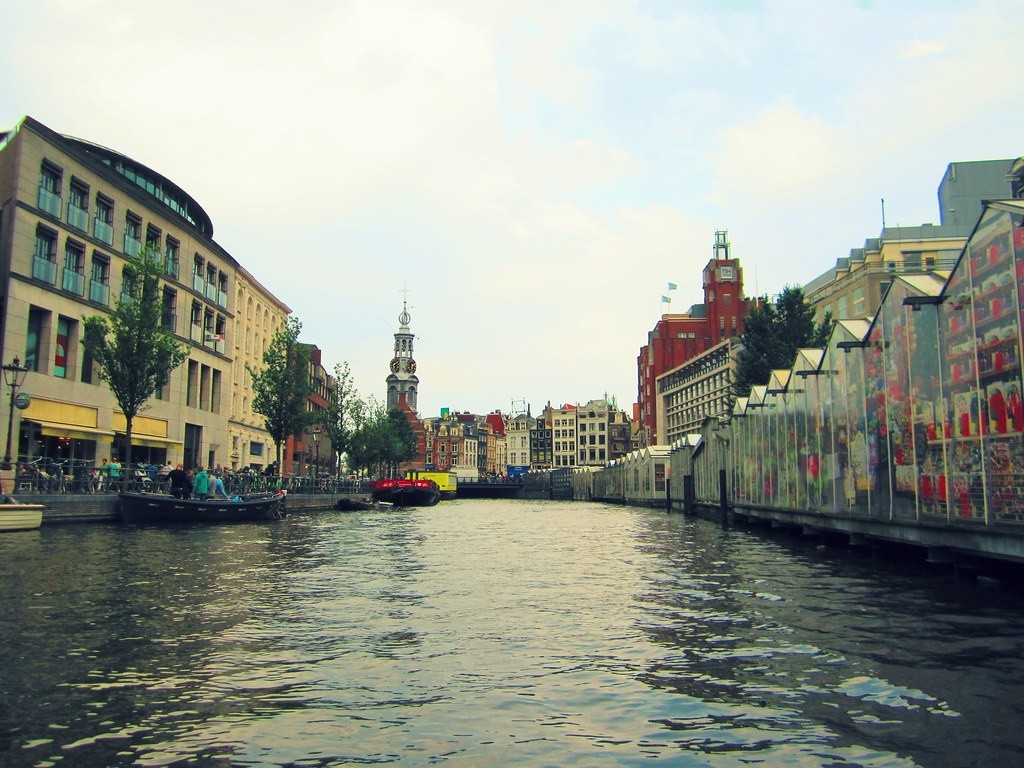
[{"x1": 26, "y1": 454, "x2": 169, "y2": 495}]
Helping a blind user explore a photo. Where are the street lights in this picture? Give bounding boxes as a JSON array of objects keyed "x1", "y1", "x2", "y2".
[
  {"x1": 0, "y1": 353, "x2": 29, "y2": 470},
  {"x1": 313, "y1": 427, "x2": 322, "y2": 491}
]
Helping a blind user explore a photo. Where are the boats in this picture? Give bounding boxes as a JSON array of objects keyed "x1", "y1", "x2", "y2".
[
  {"x1": 117, "y1": 491, "x2": 285, "y2": 525},
  {"x1": 370, "y1": 478, "x2": 440, "y2": 507},
  {"x1": 0, "y1": 497, "x2": 46, "y2": 531},
  {"x1": 337, "y1": 497, "x2": 374, "y2": 512}
]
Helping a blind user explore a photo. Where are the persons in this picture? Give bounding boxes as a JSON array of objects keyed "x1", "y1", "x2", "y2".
[
  {"x1": 16, "y1": 449, "x2": 86, "y2": 495},
  {"x1": 86, "y1": 458, "x2": 175, "y2": 496},
  {"x1": 165, "y1": 463, "x2": 187, "y2": 500},
  {"x1": 186, "y1": 460, "x2": 280, "y2": 496},
  {"x1": 320, "y1": 474, "x2": 365, "y2": 494}
]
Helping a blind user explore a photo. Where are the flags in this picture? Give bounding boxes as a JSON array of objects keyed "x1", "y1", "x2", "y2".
[{"x1": 662, "y1": 282, "x2": 677, "y2": 302}]
